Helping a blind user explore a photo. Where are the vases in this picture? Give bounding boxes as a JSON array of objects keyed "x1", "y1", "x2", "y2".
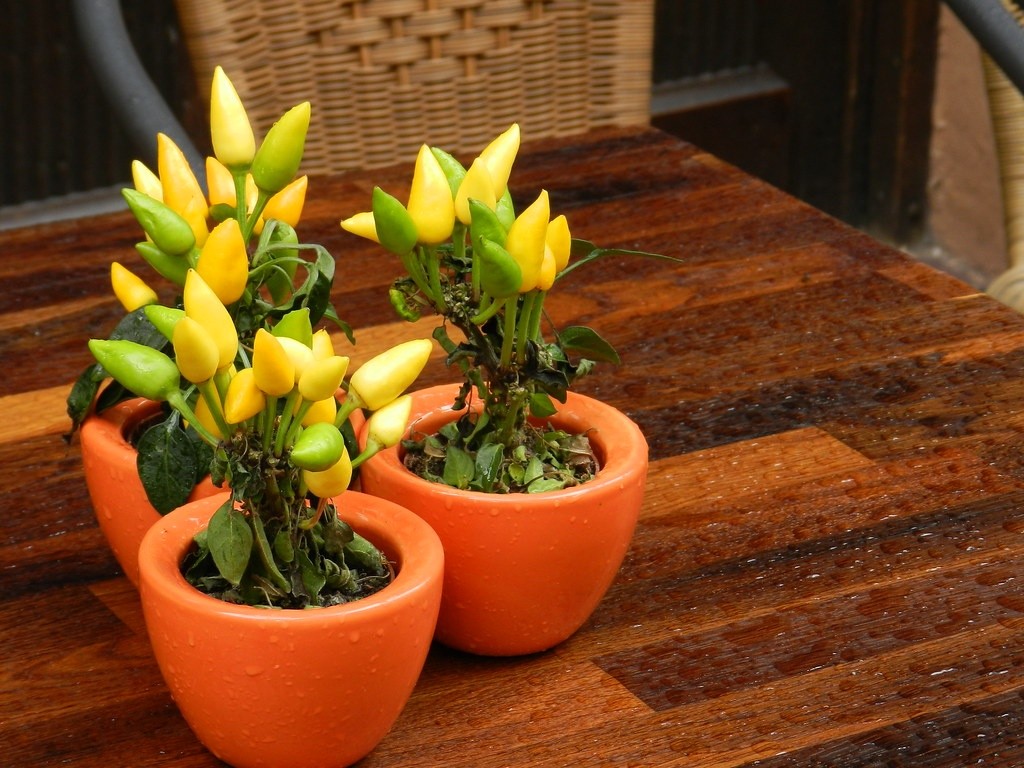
[
  {"x1": 77, "y1": 375, "x2": 368, "y2": 591},
  {"x1": 134, "y1": 488, "x2": 446, "y2": 768},
  {"x1": 359, "y1": 382, "x2": 650, "y2": 657}
]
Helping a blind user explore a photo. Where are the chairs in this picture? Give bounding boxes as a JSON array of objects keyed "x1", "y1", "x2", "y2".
[
  {"x1": 915, "y1": 0, "x2": 1024, "y2": 316},
  {"x1": 76, "y1": 0, "x2": 657, "y2": 194}
]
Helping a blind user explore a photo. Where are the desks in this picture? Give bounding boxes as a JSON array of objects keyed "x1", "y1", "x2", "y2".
[{"x1": 0, "y1": 120, "x2": 1024, "y2": 768}]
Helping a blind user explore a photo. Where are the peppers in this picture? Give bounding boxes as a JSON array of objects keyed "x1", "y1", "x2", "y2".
[{"x1": 87, "y1": 64, "x2": 572, "y2": 499}]
